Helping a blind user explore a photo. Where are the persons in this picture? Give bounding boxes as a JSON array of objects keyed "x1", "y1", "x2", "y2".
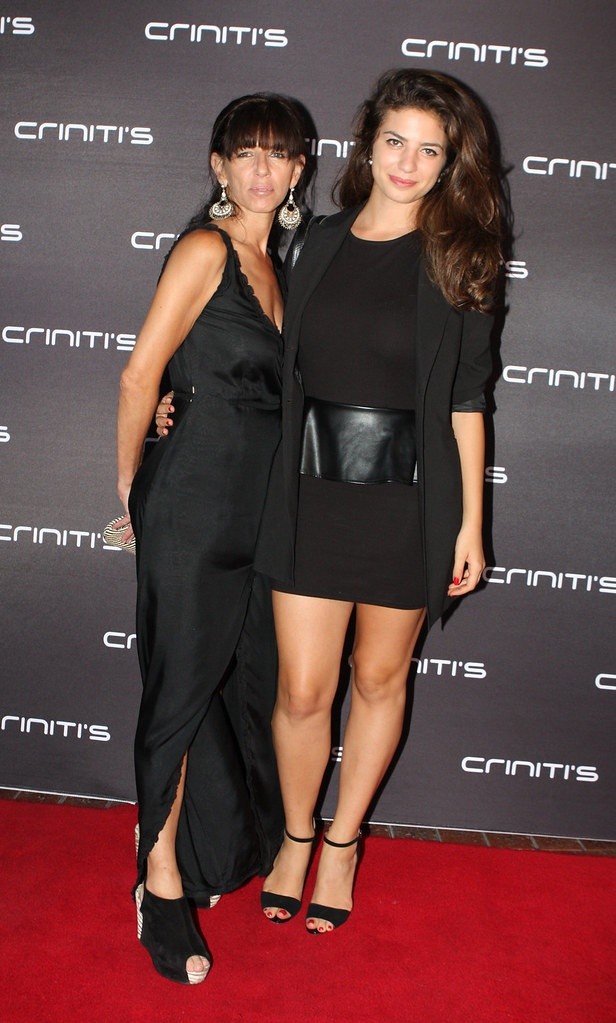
[
  {"x1": 154, "y1": 66, "x2": 515, "y2": 937},
  {"x1": 110, "y1": 90, "x2": 320, "y2": 986}
]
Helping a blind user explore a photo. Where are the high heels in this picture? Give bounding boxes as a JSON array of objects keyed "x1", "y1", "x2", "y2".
[
  {"x1": 132, "y1": 880, "x2": 213, "y2": 985},
  {"x1": 261, "y1": 817, "x2": 316, "y2": 924},
  {"x1": 134, "y1": 824, "x2": 221, "y2": 910},
  {"x1": 305, "y1": 829, "x2": 362, "y2": 934}
]
